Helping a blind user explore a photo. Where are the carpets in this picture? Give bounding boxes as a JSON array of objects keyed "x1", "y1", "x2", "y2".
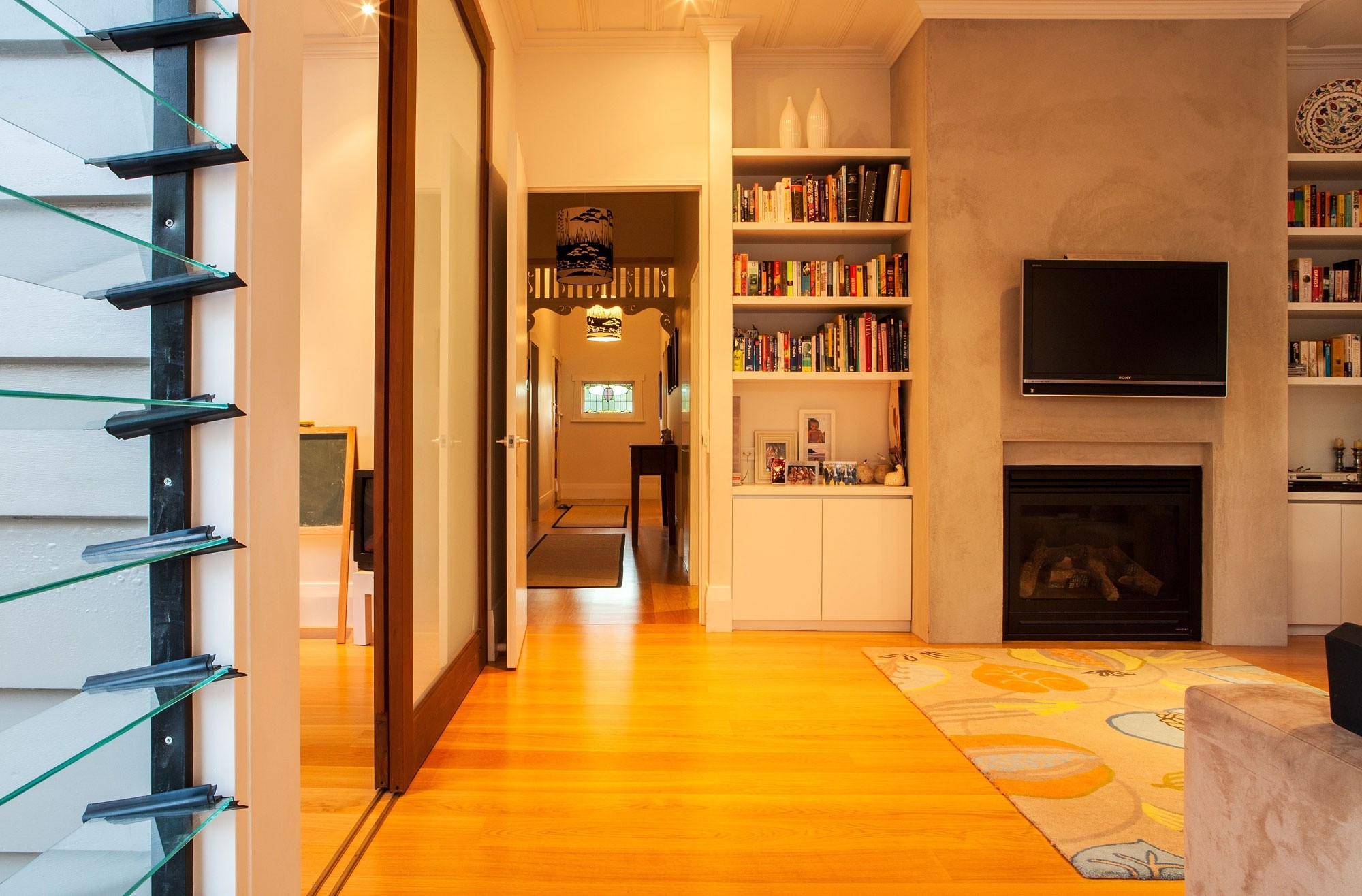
[
  {"x1": 860, "y1": 647, "x2": 1329, "y2": 875},
  {"x1": 525, "y1": 533, "x2": 626, "y2": 589},
  {"x1": 551, "y1": 504, "x2": 629, "y2": 528}
]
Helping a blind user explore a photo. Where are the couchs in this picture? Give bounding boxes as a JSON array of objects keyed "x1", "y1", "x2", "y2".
[{"x1": 1183, "y1": 620, "x2": 1362, "y2": 896}]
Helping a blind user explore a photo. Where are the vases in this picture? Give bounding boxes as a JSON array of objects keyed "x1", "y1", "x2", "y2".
[
  {"x1": 806, "y1": 88, "x2": 831, "y2": 149},
  {"x1": 780, "y1": 96, "x2": 802, "y2": 147}
]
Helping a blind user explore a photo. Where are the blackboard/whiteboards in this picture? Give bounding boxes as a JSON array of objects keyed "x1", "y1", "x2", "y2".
[{"x1": 300, "y1": 422, "x2": 357, "y2": 534}]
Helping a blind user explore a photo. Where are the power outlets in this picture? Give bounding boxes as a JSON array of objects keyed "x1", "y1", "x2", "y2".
[{"x1": 740, "y1": 446, "x2": 754, "y2": 460}]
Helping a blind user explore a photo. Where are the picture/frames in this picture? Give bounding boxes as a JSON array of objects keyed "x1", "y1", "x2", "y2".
[
  {"x1": 753, "y1": 430, "x2": 798, "y2": 484},
  {"x1": 799, "y1": 409, "x2": 836, "y2": 473},
  {"x1": 784, "y1": 460, "x2": 819, "y2": 484},
  {"x1": 658, "y1": 327, "x2": 678, "y2": 420}
]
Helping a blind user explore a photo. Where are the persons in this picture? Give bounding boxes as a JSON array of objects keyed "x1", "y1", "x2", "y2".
[
  {"x1": 814, "y1": 457, "x2": 823, "y2": 475},
  {"x1": 787, "y1": 467, "x2": 815, "y2": 485},
  {"x1": 766, "y1": 445, "x2": 785, "y2": 483},
  {"x1": 824, "y1": 464, "x2": 856, "y2": 485},
  {"x1": 808, "y1": 420, "x2": 825, "y2": 443}
]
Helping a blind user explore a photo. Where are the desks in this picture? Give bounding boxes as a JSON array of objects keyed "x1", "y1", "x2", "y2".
[{"x1": 629, "y1": 445, "x2": 679, "y2": 547}]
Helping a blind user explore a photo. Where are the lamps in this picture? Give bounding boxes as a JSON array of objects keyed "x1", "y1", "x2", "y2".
[
  {"x1": 586, "y1": 284, "x2": 623, "y2": 345},
  {"x1": 556, "y1": 191, "x2": 614, "y2": 286}
]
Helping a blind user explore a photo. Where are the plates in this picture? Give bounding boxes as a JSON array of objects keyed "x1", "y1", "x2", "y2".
[{"x1": 1295, "y1": 78, "x2": 1362, "y2": 153}]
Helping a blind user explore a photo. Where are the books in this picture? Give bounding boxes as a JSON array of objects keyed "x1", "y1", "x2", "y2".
[
  {"x1": 731, "y1": 253, "x2": 909, "y2": 296},
  {"x1": 732, "y1": 312, "x2": 910, "y2": 372},
  {"x1": 732, "y1": 163, "x2": 911, "y2": 223},
  {"x1": 1286, "y1": 183, "x2": 1362, "y2": 378}
]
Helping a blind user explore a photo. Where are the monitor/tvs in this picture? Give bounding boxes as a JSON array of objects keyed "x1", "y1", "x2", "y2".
[{"x1": 1019, "y1": 255, "x2": 1227, "y2": 399}]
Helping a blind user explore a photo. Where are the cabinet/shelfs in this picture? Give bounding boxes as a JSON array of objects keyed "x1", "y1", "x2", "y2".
[
  {"x1": 0, "y1": 0, "x2": 250, "y2": 896},
  {"x1": 739, "y1": 147, "x2": 914, "y2": 632},
  {"x1": 1288, "y1": 152, "x2": 1362, "y2": 626}
]
